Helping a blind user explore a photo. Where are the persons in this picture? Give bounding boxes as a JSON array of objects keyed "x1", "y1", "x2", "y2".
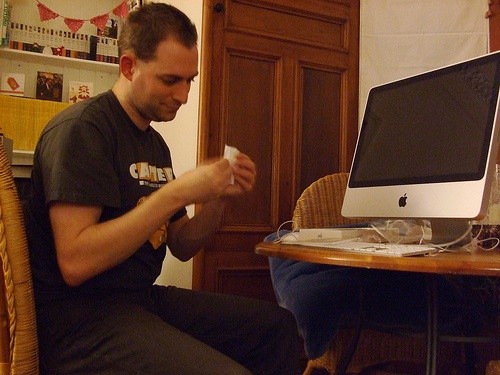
[{"x1": 26, "y1": 1, "x2": 302, "y2": 375}]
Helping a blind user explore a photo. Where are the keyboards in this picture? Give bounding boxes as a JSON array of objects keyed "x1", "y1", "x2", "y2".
[{"x1": 329, "y1": 242, "x2": 438, "y2": 257}]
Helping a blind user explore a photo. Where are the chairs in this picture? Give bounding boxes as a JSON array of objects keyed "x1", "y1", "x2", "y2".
[
  {"x1": 291, "y1": 172, "x2": 476, "y2": 375},
  {"x1": 1, "y1": 142, "x2": 39, "y2": 375}
]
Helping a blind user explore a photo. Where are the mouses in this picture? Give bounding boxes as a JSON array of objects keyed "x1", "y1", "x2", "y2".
[{"x1": 281, "y1": 232, "x2": 299, "y2": 241}]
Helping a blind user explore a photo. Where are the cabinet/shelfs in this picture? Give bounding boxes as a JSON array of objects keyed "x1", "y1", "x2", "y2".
[{"x1": 0, "y1": 47, "x2": 119, "y2": 158}]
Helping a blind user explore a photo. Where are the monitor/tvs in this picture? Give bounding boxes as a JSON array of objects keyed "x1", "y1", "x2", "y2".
[{"x1": 340, "y1": 49, "x2": 500, "y2": 253}]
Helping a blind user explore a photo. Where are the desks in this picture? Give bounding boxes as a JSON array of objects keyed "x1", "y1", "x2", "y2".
[{"x1": 254, "y1": 239, "x2": 499, "y2": 375}]
